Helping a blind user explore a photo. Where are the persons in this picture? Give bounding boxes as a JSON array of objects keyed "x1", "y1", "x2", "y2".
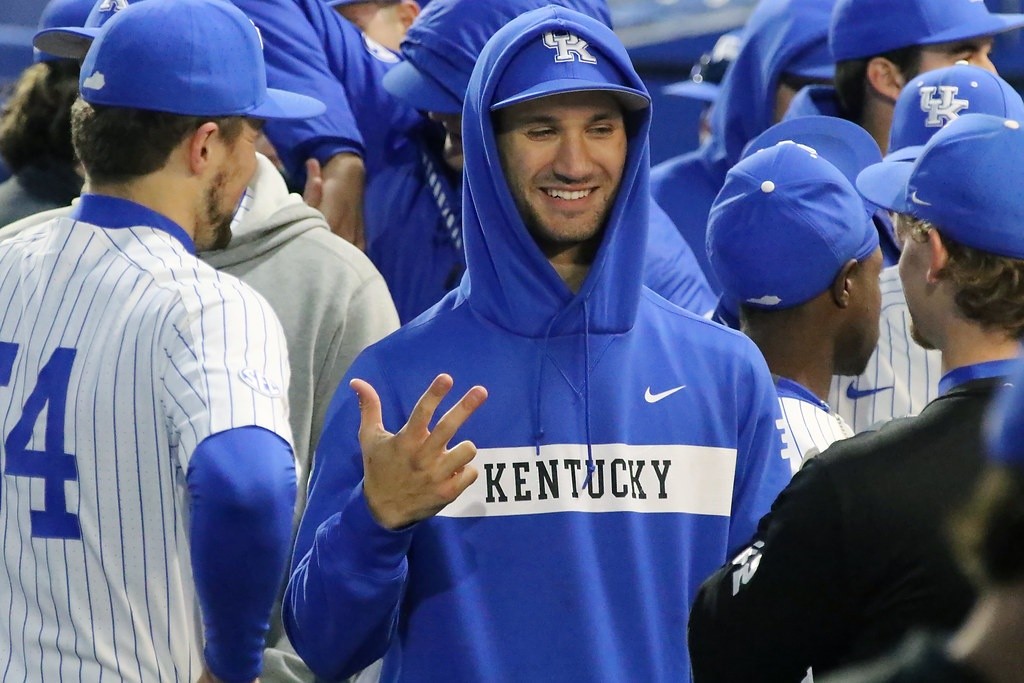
[
  {"x1": 282, "y1": 1, "x2": 792, "y2": 682},
  {"x1": 2, "y1": 2, "x2": 1024, "y2": 683},
  {"x1": 0, "y1": 1, "x2": 325, "y2": 683}
]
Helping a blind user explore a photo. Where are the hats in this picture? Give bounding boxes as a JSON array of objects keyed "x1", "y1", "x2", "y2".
[
  {"x1": 829, "y1": 0, "x2": 1024, "y2": 61},
  {"x1": 79, "y1": 0, "x2": 327, "y2": 118},
  {"x1": 491, "y1": 30, "x2": 652, "y2": 111},
  {"x1": 856, "y1": 60, "x2": 1024, "y2": 259},
  {"x1": 32, "y1": 0, "x2": 144, "y2": 60},
  {"x1": 662, "y1": 31, "x2": 743, "y2": 102},
  {"x1": 706, "y1": 117, "x2": 882, "y2": 309},
  {"x1": 784, "y1": 39, "x2": 834, "y2": 78}
]
